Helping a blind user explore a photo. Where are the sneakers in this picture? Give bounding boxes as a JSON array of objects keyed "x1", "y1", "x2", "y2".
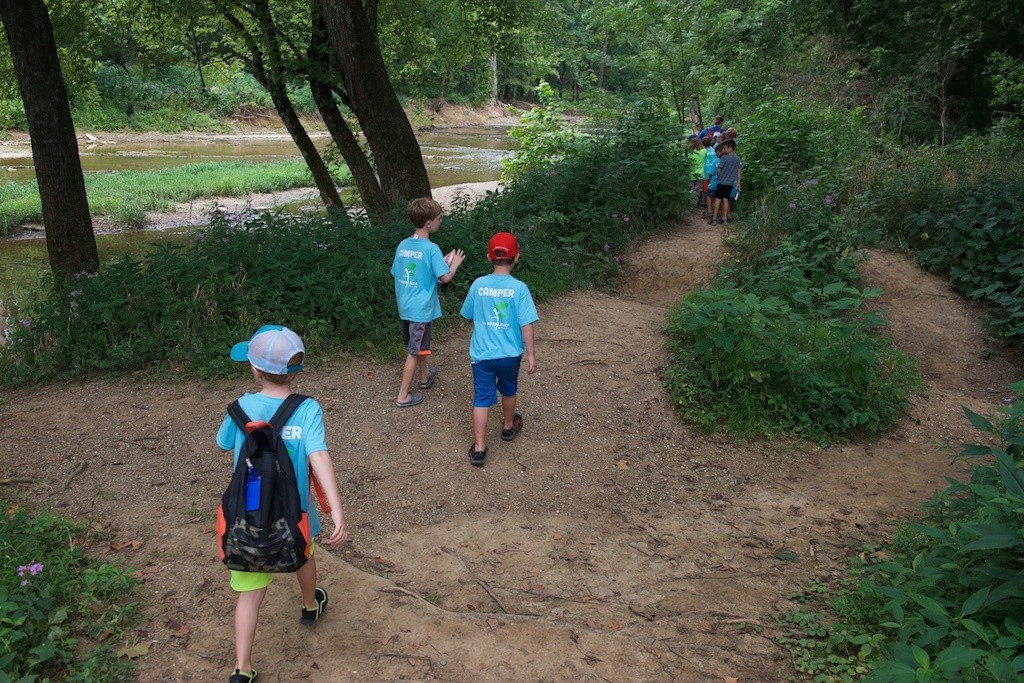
[
  {"x1": 229, "y1": 666, "x2": 259, "y2": 683},
  {"x1": 501, "y1": 414, "x2": 523, "y2": 440},
  {"x1": 469, "y1": 443, "x2": 488, "y2": 465},
  {"x1": 300, "y1": 587, "x2": 328, "y2": 626}
]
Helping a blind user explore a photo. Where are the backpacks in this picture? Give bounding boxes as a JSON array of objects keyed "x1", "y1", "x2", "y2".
[{"x1": 217, "y1": 393, "x2": 329, "y2": 574}]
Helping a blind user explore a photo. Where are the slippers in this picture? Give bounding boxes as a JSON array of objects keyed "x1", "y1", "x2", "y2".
[
  {"x1": 396, "y1": 391, "x2": 423, "y2": 406},
  {"x1": 419, "y1": 367, "x2": 436, "y2": 388}
]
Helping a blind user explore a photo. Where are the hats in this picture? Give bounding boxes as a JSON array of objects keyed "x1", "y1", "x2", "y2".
[
  {"x1": 231, "y1": 325, "x2": 305, "y2": 375},
  {"x1": 489, "y1": 232, "x2": 517, "y2": 259}
]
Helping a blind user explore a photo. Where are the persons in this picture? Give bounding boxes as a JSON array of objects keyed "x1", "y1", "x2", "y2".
[
  {"x1": 461, "y1": 232, "x2": 538, "y2": 463},
  {"x1": 201, "y1": 323, "x2": 347, "y2": 683},
  {"x1": 390, "y1": 198, "x2": 466, "y2": 407},
  {"x1": 684, "y1": 116, "x2": 742, "y2": 225}
]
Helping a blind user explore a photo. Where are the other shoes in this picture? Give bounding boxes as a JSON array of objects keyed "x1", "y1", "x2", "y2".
[
  {"x1": 719, "y1": 219, "x2": 726, "y2": 224},
  {"x1": 708, "y1": 220, "x2": 717, "y2": 225},
  {"x1": 699, "y1": 201, "x2": 707, "y2": 208},
  {"x1": 703, "y1": 212, "x2": 713, "y2": 217},
  {"x1": 727, "y1": 215, "x2": 733, "y2": 221}
]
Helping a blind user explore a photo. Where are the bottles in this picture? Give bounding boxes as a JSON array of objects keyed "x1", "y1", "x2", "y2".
[{"x1": 734, "y1": 189, "x2": 740, "y2": 200}]
